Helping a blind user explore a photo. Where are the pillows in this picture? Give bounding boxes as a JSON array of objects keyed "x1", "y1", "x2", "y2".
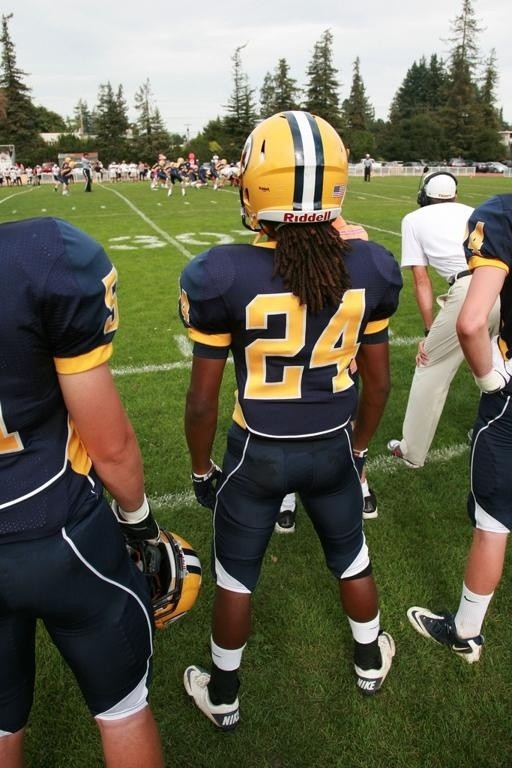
[
  {"x1": 423, "y1": 172, "x2": 457, "y2": 200},
  {"x1": 152, "y1": 528, "x2": 202, "y2": 630},
  {"x1": 238, "y1": 111, "x2": 349, "y2": 231}
]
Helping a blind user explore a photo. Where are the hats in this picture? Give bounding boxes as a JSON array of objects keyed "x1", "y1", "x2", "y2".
[
  {"x1": 1, "y1": 166, "x2": 25, "y2": 175},
  {"x1": 42, "y1": 160, "x2": 94, "y2": 178},
  {"x1": 370, "y1": 156, "x2": 512, "y2": 174}
]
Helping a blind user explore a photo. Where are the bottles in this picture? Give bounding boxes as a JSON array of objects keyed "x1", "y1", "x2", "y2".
[{"x1": 111, "y1": 493, "x2": 160, "y2": 547}]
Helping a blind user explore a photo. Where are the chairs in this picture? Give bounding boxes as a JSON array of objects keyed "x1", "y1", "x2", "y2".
[
  {"x1": 407, "y1": 606, "x2": 485, "y2": 665},
  {"x1": 355, "y1": 631, "x2": 396, "y2": 695},
  {"x1": 183, "y1": 665, "x2": 240, "y2": 732},
  {"x1": 274, "y1": 503, "x2": 297, "y2": 535},
  {"x1": 362, "y1": 488, "x2": 378, "y2": 520},
  {"x1": 387, "y1": 439, "x2": 425, "y2": 469}
]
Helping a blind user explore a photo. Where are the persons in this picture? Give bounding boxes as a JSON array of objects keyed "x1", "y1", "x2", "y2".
[
  {"x1": 1, "y1": 215, "x2": 204, "y2": 768},
  {"x1": 406, "y1": 195, "x2": 509, "y2": 660},
  {"x1": 0, "y1": 156, "x2": 240, "y2": 199},
  {"x1": 362, "y1": 153, "x2": 373, "y2": 183},
  {"x1": 182, "y1": 108, "x2": 397, "y2": 730},
  {"x1": 273, "y1": 417, "x2": 379, "y2": 530},
  {"x1": 387, "y1": 170, "x2": 505, "y2": 472}
]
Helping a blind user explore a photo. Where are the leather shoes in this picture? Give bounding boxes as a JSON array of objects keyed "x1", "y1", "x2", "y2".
[
  {"x1": 472, "y1": 335, "x2": 512, "y2": 394},
  {"x1": 352, "y1": 448, "x2": 368, "y2": 480},
  {"x1": 191, "y1": 459, "x2": 222, "y2": 509}
]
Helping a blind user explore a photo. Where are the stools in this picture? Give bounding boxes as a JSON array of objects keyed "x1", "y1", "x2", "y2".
[{"x1": 449, "y1": 270, "x2": 473, "y2": 286}]
